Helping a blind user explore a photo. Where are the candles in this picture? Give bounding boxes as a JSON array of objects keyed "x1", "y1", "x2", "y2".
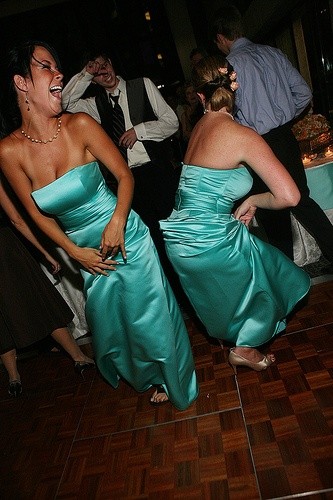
[
  {"x1": 324, "y1": 150, "x2": 333, "y2": 157},
  {"x1": 303, "y1": 157, "x2": 311, "y2": 164}
]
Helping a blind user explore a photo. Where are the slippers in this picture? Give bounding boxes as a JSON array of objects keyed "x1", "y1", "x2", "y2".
[{"x1": 148, "y1": 384, "x2": 170, "y2": 406}]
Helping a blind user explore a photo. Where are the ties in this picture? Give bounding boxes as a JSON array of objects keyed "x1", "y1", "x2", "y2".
[{"x1": 109, "y1": 90, "x2": 128, "y2": 166}]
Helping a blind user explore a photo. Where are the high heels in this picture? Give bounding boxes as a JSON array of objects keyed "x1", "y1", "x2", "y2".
[{"x1": 229, "y1": 349, "x2": 275, "y2": 375}]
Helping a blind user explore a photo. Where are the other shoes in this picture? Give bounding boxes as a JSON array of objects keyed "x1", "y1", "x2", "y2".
[
  {"x1": 8, "y1": 371, "x2": 23, "y2": 399},
  {"x1": 302, "y1": 255, "x2": 333, "y2": 278},
  {"x1": 73, "y1": 358, "x2": 97, "y2": 391}
]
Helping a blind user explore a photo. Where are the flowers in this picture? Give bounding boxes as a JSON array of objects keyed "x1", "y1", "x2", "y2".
[
  {"x1": 230, "y1": 82, "x2": 239, "y2": 92},
  {"x1": 229, "y1": 71, "x2": 237, "y2": 82},
  {"x1": 219, "y1": 67, "x2": 228, "y2": 75}
]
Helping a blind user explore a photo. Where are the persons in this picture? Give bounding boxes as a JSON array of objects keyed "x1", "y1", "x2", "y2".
[
  {"x1": 0, "y1": 180, "x2": 90, "y2": 396},
  {"x1": 211, "y1": 15, "x2": 333, "y2": 277},
  {"x1": 0, "y1": 41, "x2": 200, "y2": 411},
  {"x1": 171, "y1": 47, "x2": 209, "y2": 171},
  {"x1": 62, "y1": 51, "x2": 188, "y2": 304},
  {"x1": 157, "y1": 54, "x2": 312, "y2": 373}
]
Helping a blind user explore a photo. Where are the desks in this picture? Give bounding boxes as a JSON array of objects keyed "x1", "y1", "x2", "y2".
[{"x1": 289, "y1": 149, "x2": 333, "y2": 267}]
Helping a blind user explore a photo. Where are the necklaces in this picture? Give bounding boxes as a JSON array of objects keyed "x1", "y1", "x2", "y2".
[{"x1": 21, "y1": 115, "x2": 62, "y2": 144}]
